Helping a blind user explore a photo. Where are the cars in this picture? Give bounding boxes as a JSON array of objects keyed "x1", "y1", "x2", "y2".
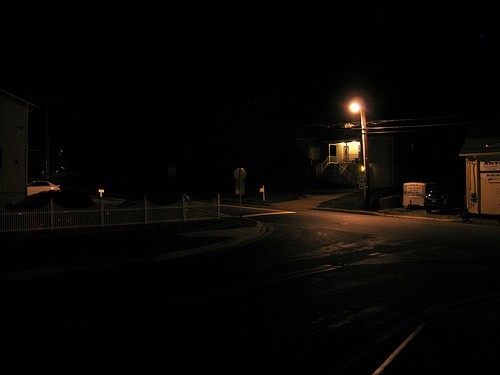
[
  {"x1": 27, "y1": 180, "x2": 61, "y2": 197},
  {"x1": 423, "y1": 190, "x2": 449, "y2": 213}
]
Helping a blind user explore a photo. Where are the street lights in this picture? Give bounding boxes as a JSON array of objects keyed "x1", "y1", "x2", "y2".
[{"x1": 349, "y1": 101, "x2": 367, "y2": 201}]
